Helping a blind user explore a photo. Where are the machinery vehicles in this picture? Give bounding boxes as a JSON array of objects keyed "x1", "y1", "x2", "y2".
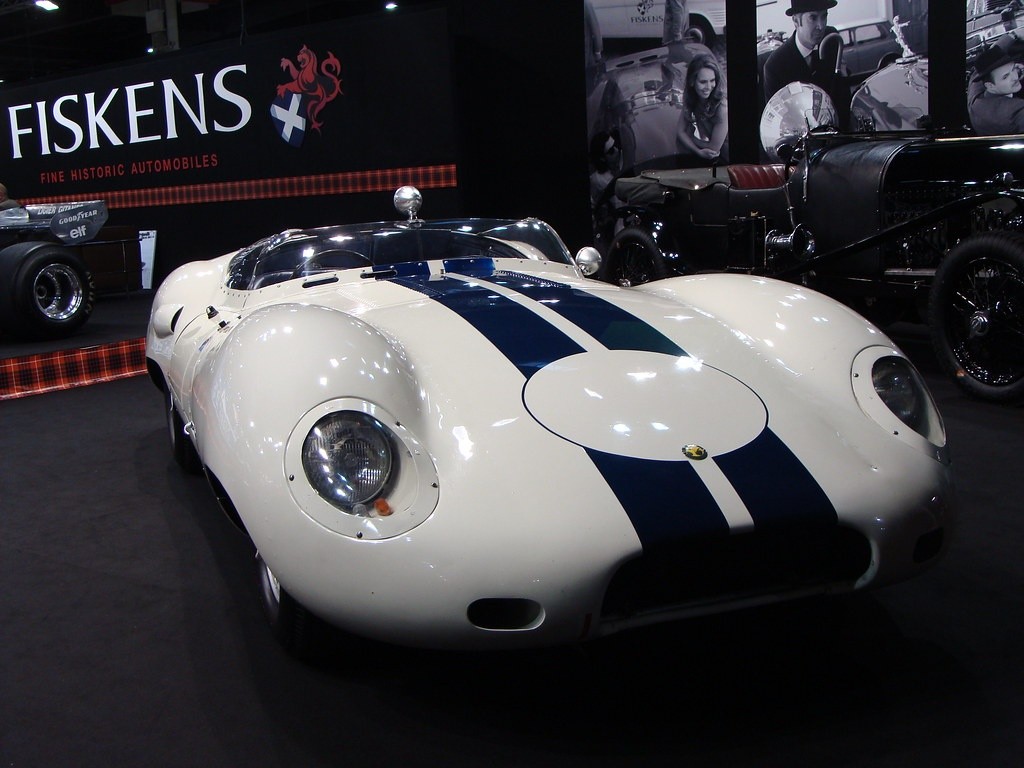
[{"x1": 603, "y1": 108, "x2": 1024, "y2": 406}]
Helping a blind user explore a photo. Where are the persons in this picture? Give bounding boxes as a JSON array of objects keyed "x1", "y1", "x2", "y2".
[
  {"x1": 967, "y1": 45, "x2": 1024, "y2": 135},
  {"x1": 763, "y1": 0, "x2": 853, "y2": 135},
  {"x1": 657, "y1": 1, "x2": 728, "y2": 167}
]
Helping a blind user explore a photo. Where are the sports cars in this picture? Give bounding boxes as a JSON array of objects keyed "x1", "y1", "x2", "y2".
[
  {"x1": 143, "y1": 183, "x2": 952, "y2": 756},
  {"x1": 0, "y1": 186, "x2": 110, "y2": 345}
]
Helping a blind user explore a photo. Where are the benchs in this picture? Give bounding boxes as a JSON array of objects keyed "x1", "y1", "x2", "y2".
[{"x1": 728, "y1": 163, "x2": 798, "y2": 190}]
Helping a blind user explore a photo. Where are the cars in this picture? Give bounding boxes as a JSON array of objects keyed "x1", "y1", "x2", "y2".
[{"x1": 598, "y1": 19, "x2": 1023, "y2": 114}]
